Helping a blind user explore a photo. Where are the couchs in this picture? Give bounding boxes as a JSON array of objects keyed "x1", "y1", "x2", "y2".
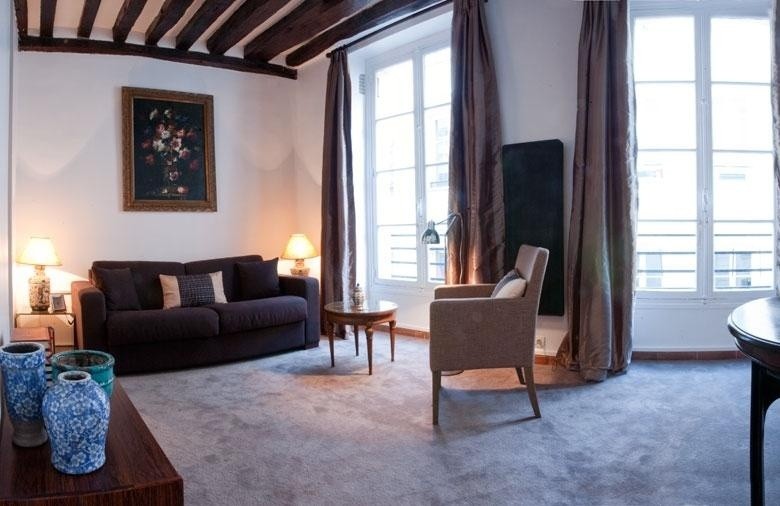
[{"x1": 71, "y1": 254, "x2": 322, "y2": 376}]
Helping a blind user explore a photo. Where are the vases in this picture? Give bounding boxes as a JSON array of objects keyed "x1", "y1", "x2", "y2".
[
  {"x1": 50, "y1": 350, "x2": 115, "y2": 400},
  {"x1": 42, "y1": 370, "x2": 111, "y2": 475},
  {"x1": 0, "y1": 342, "x2": 48, "y2": 446}
]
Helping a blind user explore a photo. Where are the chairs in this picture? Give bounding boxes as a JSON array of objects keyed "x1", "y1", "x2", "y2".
[{"x1": 428, "y1": 243, "x2": 550, "y2": 426}]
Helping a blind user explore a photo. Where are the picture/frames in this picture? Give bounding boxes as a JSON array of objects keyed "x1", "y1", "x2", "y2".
[{"x1": 119, "y1": 85, "x2": 218, "y2": 214}]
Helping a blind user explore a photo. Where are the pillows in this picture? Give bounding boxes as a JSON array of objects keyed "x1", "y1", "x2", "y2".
[
  {"x1": 90, "y1": 263, "x2": 141, "y2": 311},
  {"x1": 158, "y1": 270, "x2": 230, "y2": 311},
  {"x1": 231, "y1": 257, "x2": 281, "y2": 302},
  {"x1": 488, "y1": 268, "x2": 528, "y2": 300}
]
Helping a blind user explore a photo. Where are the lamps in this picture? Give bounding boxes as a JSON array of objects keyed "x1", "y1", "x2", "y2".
[
  {"x1": 279, "y1": 231, "x2": 323, "y2": 278},
  {"x1": 420, "y1": 210, "x2": 466, "y2": 284},
  {"x1": 14, "y1": 232, "x2": 66, "y2": 313}
]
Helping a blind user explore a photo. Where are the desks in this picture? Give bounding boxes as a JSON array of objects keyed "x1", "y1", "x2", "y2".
[
  {"x1": 725, "y1": 290, "x2": 780, "y2": 505},
  {"x1": 1, "y1": 371, "x2": 185, "y2": 505}
]
